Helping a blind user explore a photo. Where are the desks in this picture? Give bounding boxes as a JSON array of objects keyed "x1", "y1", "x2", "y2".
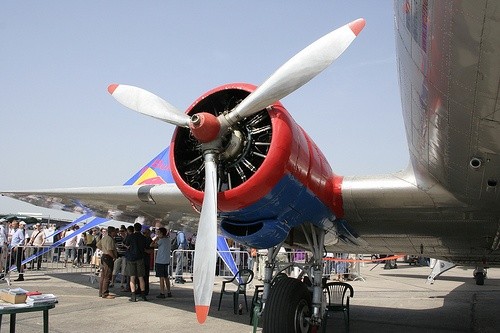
[{"x1": 0, "y1": 299, "x2": 55, "y2": 333}]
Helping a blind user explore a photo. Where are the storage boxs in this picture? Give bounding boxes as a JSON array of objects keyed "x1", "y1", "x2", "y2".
[{"x1": 0, "y1": 290, "x2": 27, "y2": 304}]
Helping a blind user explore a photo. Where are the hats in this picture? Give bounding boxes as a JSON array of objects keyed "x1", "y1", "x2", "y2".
[
  {"x1": 36, "y1": 222, "x2": 41, "y2": 226},
  {"x1": 19, "y1": 221, "x2": 26, "y2": 225}
]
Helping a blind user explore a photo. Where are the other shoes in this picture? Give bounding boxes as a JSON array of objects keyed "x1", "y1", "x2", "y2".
[
  {"x1": 156, "y1": 293, "x2": 165, "y2": 298},
  {"x1": 14, "y1": 274, "x2": 24, "y2": 281},
  {"x1": 27, "y1": 266, "x2": 34, "y2": 270},
  {"x1": 99, "y1": 294, "x2": 117, "y2": 299},
  {"x1": 128, "y1": 292, "x2": 137, "y2": 302},
  {"x1": 120, "y1": 284, "x2": 128, "y2": 292},
  {"x1": 168, "y1": 292, "x2": 173, "y2": 297},
  {"x1": 109, "y1": 283, "x2": 115, "y2": 288},
  {"x1": 140, "y1": 290, "x2": 148, "y2": 301},
  {"x1": 175, "y1": 279, "x2": 186, "y2": 284}
]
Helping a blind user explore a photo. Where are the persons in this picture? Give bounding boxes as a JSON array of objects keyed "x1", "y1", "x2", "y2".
[
  {"x1": 126, "y1": 223, "x2": 148, "y2": 302},
  {"x1": 96, "y1": 226, "x2": 117, "y2": 299},
  {"x1": 53, "y1": 225, "x2": 197, "y2": 288},
  {"x1": 216, "y1": 239, "x2": 355, "y2": 281},
  {"x1": 0, "y1": 218, "x2": 29, "y2": 281},
  {"x1": 150, "y1": 227, "x2": 172, "y2": 298},
  {"x1": 27, "y1": 222, "x2": 46, "y2": 270}
]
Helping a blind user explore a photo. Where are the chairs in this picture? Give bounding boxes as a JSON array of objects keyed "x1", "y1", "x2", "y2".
[
  {"x1": 217, "y1": 269, "x2": 254, "y2": 314},
  {"x1": 249, "y1": 286, "x2": 264, "y2": 333},
  {"x1": 322, "y1": 281, "x2": 354, "y2": 333}
]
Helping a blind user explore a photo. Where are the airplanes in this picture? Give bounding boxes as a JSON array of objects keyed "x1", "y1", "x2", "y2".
[{"x1": 1, "y1": 0, "x2": 500, "y2": 333}]
